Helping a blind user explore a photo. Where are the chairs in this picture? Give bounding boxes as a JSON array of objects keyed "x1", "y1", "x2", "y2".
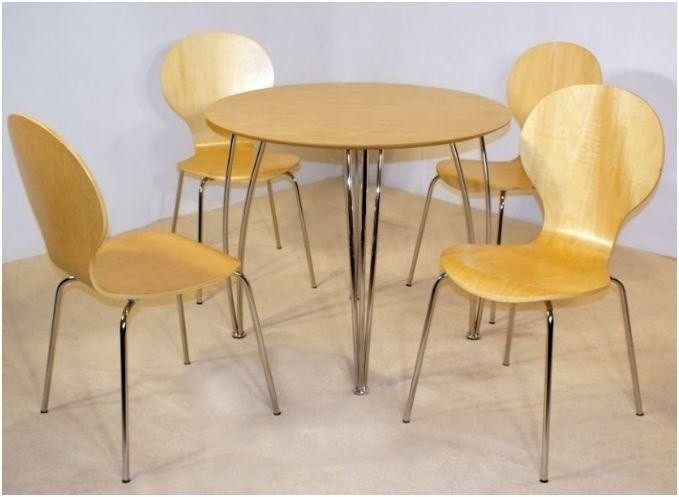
[
  {"x1": 403, "y1": 84, "x2": 665, "y2": 480},
  {"x1": 6, "y1": 112, "x2": 281, "y2": 483},
  {"x1": 158, "y1": 30, "x2": 317, "y2": 291},
  {"x1": 407, "y1": 42, "x2": 604, "y2": 324}
]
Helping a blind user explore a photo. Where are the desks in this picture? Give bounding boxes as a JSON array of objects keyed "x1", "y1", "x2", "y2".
[{"x1": 205, "y1": 80, "x2": 517, "y2": 394}]
